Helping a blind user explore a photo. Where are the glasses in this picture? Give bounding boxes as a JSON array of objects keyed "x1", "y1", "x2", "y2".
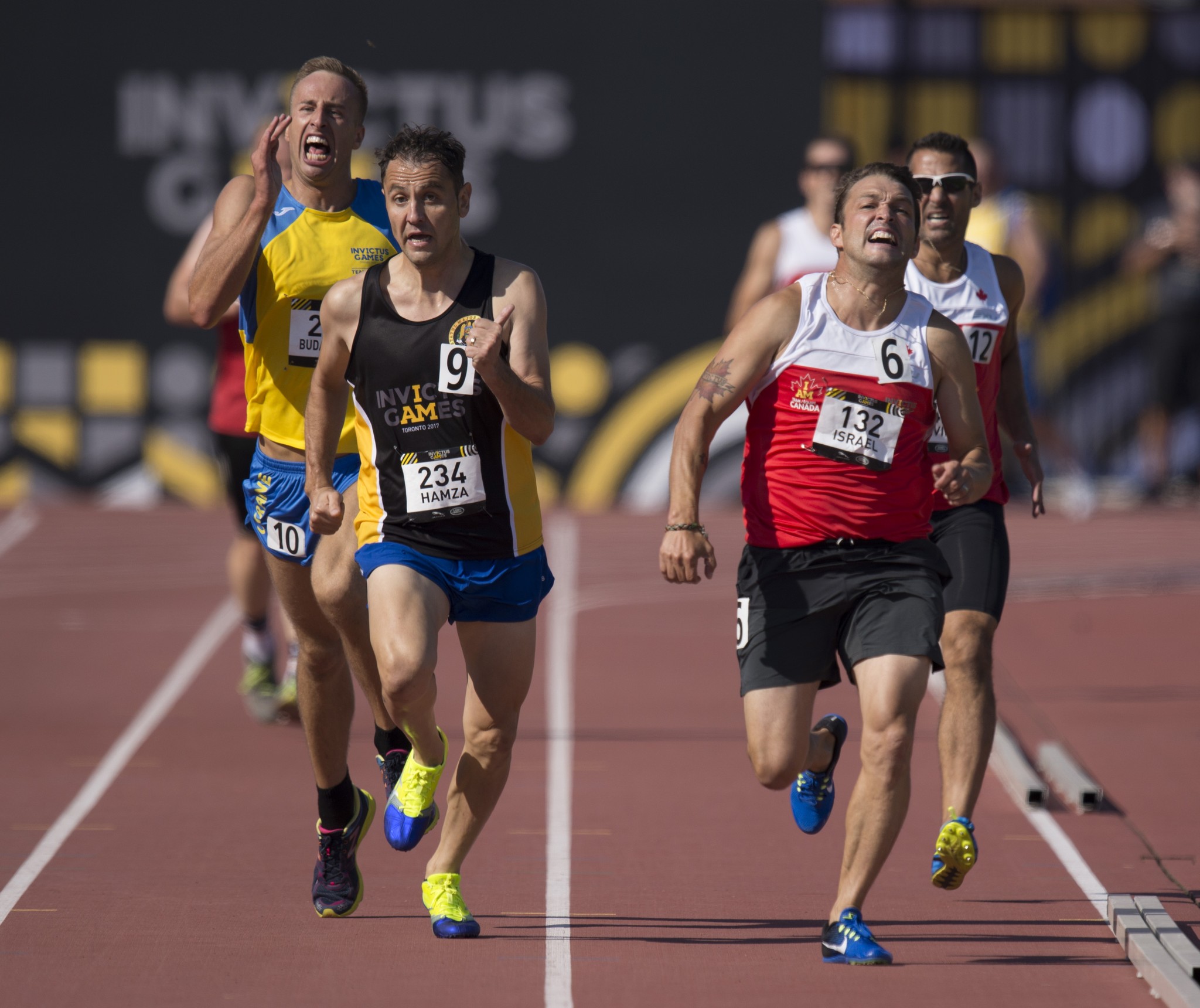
[{"x1": 912, "y1": 173, "x2": 975, "y2": 193}]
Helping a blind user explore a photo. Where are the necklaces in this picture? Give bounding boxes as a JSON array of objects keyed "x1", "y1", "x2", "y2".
[{"x1": 829, "y1": 271, "x2": 905, "y2": 316}]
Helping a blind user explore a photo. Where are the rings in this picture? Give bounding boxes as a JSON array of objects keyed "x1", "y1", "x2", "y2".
[
  {"x1": 963, "y1": 485, "x2": 969, "y2": 492},
  {"x1": 470, "y1": 337, "x2": 476, "y2": 346}
]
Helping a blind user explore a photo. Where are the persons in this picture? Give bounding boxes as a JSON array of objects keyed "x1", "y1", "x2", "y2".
[
  {"x1": 657, "y1": 130, "x2": 1042, "y2": 966},
  {"x1": 1123, "y1": 151, "x2": 1200, "y2": 512},
  {"x1": 167, "y1": 56, "x2": 558, "y2": 940}
]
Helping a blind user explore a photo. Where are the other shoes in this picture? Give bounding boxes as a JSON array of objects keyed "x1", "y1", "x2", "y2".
[
  {"x1": 241, "y1": 663, "x2": 276, "y2": 723},
  {"x1": 277, "y1": 675, "x2": 299, "y2": 719}
]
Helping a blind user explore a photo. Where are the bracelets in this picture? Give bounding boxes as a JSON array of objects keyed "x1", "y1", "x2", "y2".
[{"x1": 663, "y1": 524, "x2": 707, "y2": 539}]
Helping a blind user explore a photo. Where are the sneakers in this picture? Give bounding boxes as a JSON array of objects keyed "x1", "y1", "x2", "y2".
[
  {"x1": 383, "y1": 725, "x2": 448, "y2": 852},
  {"x1": 311, "y1": 783, "x2": 376, "y2": 917},
  {"x1": 821, "y1": 907, "x2": 893, "y2": 965},
  {"x1": 422, "y1": 873, "x2": 480, "y2": 938},
  {"x1": 931, "y1": 806, "x2": 978, "y2": 890},
  {"x1": 375, "y1": 749, "x2": 440, "y2": 835},
  {"x1": 790, "y1": 713, "x2": 848, "y2": 835}
]
